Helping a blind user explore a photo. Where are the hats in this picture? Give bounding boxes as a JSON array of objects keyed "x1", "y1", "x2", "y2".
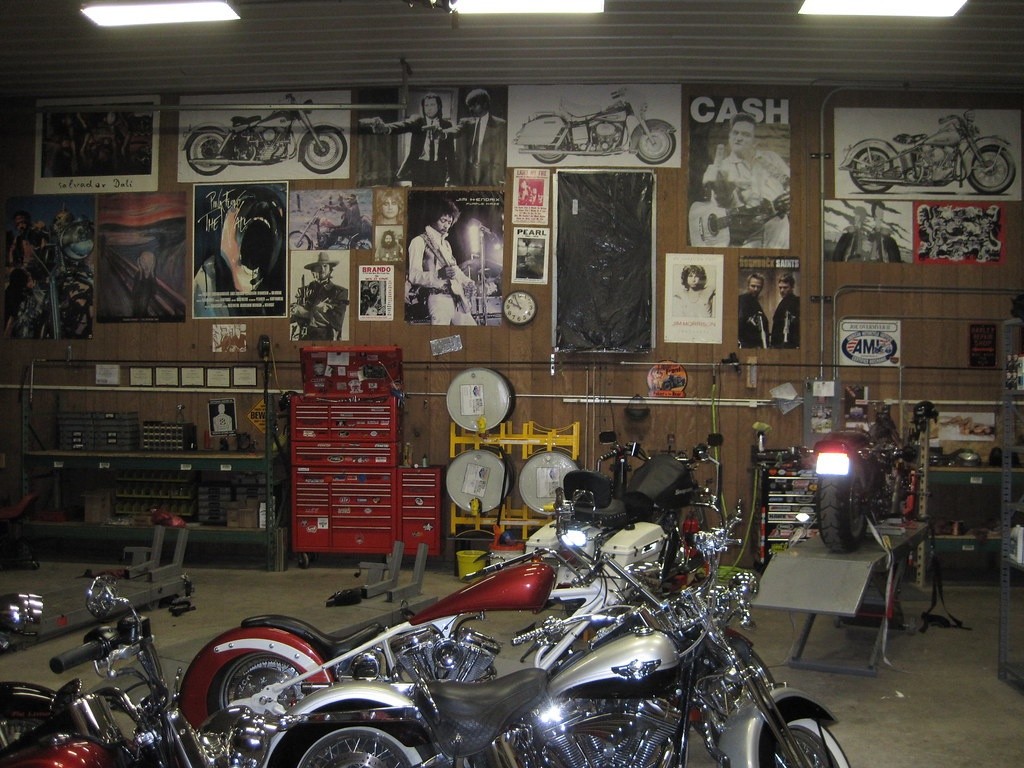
[{"x1": 305, "y1": 252, "x2": 339, "y2": 269}]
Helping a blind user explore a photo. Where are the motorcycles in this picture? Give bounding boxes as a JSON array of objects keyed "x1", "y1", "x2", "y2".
[
  {"x1": 182, "y1": 90, "x2": 348, "y2": 175},
  {"x1": 514, "y1": 91, "x2": 676, "y2": 164},
  {"x1": 843, "y1": 110, "x2": 1016, "y2": 195},
  {"x1": 0, "y1": 430, "x2": 849, "y2": 768},
  {"x1": 812, "y1": 401, "x2": 941, "y2": 554},
  {"x1": 288, "y1": 204, "x2": 373, "y2": 251}
]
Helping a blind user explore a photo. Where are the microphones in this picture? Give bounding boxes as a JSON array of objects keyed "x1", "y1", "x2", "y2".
[{"x1": 468, "y1": 218, "x2": 491, "y2": 235}]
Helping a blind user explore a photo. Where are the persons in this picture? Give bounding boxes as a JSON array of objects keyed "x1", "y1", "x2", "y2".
[
  {"x1": 431, "y1": 90, "x2": 507, "y2": 186},
  {"x1": 289, "y1": 250, "x2": 348, "y2": 342},
  {"x1": 700, "y1": 113, "x2": 790, "y2": 249},
  {"x1": 941, "y1": 414, "x2": 994, "y2": 437},
  {"x1": 371, "y1": 91, "x2": 457, "y2": 187},
  {"x1": 671, "y1": 262, "x2": 717, "y2": 320},
  {"x1": 407, "y1": 200, "x2": 479, "y2": 326},
  {"x1": 517, "y1": 179, "x2": 544, "y2": 207},
  {"x1": 376, "y1": 231, "x2": 403, "y2": 262},
  {"x1": 736, "y1": 274, "x2": 770, "y2": 347},
  {"x1": 3, "y1": 213, "x2": 49, "y2": 338},
  {"x1": 768, "y1": 274, "x2": 799, "y2": 348},
  {"x1": 377, "y1": 189, "x2": 405, "y2": 224},
  {"x1": 316, "y1": 191, "x2": 362, "y2": 250},
  {"x1": 361, "y1": 282, "x2": 378, "y2": 316}
]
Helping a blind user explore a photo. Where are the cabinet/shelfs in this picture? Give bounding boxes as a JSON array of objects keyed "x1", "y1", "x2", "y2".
[
  {"x1": 19, "y1": 384, "x2": 292, "y2": 570},
  {"x1": 901, "y1": 408, "x2": 1023, "y2": 589},
  {"x1": 999, "y1": 317, "x2": 1024, "y2": 698},
  {"x1": 753, "y1": 462, "x2": 817, "y2": 578},
  {"x1": 290, "y1": 344, "x2": 447, "y2": 568}
]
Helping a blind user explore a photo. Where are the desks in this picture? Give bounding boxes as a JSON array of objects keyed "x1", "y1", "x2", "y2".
[{"x1": 751, "y1": 518, "x2": 929, "y2": 676}]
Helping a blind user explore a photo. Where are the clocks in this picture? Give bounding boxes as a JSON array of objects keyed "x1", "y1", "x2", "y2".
[{"x1": 502, "y1": 291, "x2": 537, "y2": 325}]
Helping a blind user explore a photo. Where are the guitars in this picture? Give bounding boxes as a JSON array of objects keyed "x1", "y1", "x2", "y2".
[
  {"x1": 687, "y1": 192, "x2": 791, "y2": 247},
  {"x1": 425, "y1": 224, "x2": 473, "y2": 313}
]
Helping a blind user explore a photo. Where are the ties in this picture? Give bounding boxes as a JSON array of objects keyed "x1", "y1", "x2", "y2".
[
  {"x1": 429, "y1": 120, "x2": 436, "y2": 162},
  {"x1": 472, "y1": 117, "x2": 481, "y2": 164}
]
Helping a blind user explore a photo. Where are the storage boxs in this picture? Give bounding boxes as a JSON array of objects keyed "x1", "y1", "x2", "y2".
[
  {"x1": 600, "y1": 522, "x2": 667, "y2": 595},
  {"x1": 526, "y1": 518, "x2": 560, "y2": 558}
]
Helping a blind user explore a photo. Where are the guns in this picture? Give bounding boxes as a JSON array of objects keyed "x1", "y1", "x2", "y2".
[
  {"x1": 420, "y1": 123, "x2": 439, "y2": 138},
  {"x1": 358, "y1": 116, "x2": 382, "y2": 133}
]
